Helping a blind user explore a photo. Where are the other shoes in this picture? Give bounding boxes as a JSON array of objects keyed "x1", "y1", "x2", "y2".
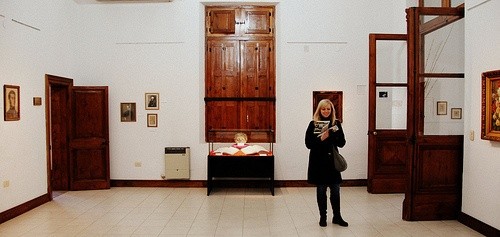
[
  {"x1": 320, "y1": 215, "x2": 327, "y2": 226},
  {"x1": 333, "y1": 216, "x2": 348, "y2": 226}
]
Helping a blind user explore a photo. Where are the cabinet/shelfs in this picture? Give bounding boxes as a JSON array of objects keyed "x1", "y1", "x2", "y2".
[{"x1": 204, "y1": 5, "x2": 275, "y2": 144}]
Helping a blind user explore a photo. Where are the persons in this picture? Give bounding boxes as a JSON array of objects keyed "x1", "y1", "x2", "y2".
[{"x1": 305, "y1": 99, "x2": 347, "y2": 227}]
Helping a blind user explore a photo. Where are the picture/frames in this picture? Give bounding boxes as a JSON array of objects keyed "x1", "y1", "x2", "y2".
[
  {"x1": 451, "y1": 108, "x2": 462, "y2": 119},
  {"x1": 481, "y1": 70, "x2": 500, "y2": 142},
  {"x1": 437, "y1": 101, "x2": 447, "y2": 115},
  {"x1": 313, "y1": 91, "x2": 343, "y2": 123},
  {"x1": 147, "y1": 114, "x2": 157, "y2": 127},
  {"x1": 4, "y1": 85, "x2": 20, "y2": 121},
  {"x1": 145, "y1": 93, "x2": 159, "y2": 110},
  {"x1": 121, "y1": 103, "x2": 136, "y2": 122}
]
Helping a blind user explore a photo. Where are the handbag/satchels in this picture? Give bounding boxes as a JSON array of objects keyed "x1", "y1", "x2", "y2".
[{"x1": 333, "y1": 146, "x2": 347, "y2": 172}]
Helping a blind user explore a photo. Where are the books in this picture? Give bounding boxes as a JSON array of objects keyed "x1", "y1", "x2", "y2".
[
  {"x1": 314, "y1": 120, "x2": 330, "y2": 135},
  {"x1": 214, "y1": 145, "x2": 269, "y2": 155}
]
